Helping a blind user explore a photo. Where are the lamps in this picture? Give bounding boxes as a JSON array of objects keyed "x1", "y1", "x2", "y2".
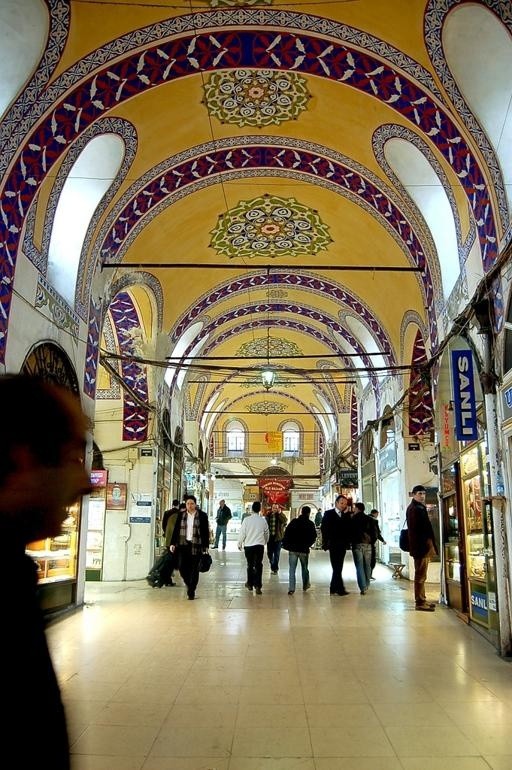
[
  {"x1": 271, "y1": 457, "x2": 276, "y2": 464},
  {"x1": 261, "y1": 326, "x2": 277, "y2": 391}
]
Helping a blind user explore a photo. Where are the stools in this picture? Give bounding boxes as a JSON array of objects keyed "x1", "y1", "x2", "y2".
[{"x1": 389, "y1": 562, "x2": 406, "y2": 578}]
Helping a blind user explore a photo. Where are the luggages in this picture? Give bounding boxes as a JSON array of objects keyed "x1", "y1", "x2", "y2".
[{"x1": 146, "y1": 551, "x2": 175, "y2": 589}]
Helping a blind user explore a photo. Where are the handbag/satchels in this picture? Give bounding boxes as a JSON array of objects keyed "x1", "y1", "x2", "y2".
[
  {"x1": 197, "y1": 553, "x2": 212, "y2": 573},
  {"x1": 400, "y1": 529, "x2": 411, "y2": 552}
]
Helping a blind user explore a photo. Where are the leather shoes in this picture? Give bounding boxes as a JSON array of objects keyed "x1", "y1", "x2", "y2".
[
  {"x1": 426, "y1": 602, "x2": 435, "y2": 607},
  {"x1": 255, "y1": 588, "x2": 262, "y2": 594},
  {"x1": 287, "y1": 591, "x2": 294, "y2": 595},
  {"x1": 303, "y1": 583, "x2": 311, "y2": 591},
  {"x1": 244, "y1": 582, "x2": 253, "y2": 590},
  {"x1": 416, "y1": 604, "x2": 435, "y2": 611}
]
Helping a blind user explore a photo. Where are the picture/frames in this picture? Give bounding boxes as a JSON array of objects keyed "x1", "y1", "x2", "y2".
[{"x1": 107, "y1": 482, "x2": 128, "y2": 510}]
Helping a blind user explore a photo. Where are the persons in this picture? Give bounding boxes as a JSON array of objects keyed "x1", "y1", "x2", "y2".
[
  {"x1": 211, "y1": 500, "x2": 231, "y2": 550},
  {"x1": 162, "y1": 494, "x2": 209, "y2": 600},
  {"x1": 406, "y1": 484, "x2": 440, "y2": 611},
  {"x1": 237, "y1": 495, "x2": 387, "y2": 596},
  {"x1": 0, "y1": 372, "x2": 94, "y2": 768}
]
